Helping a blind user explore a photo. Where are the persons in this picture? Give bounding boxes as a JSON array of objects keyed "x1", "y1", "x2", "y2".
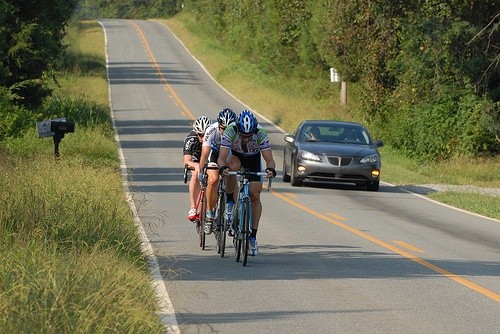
[
  {"x1": 218, "y1": 108, "x2": 277, "y2": 256},
  {"x1": 307, "y1": 127, "x2": 322, "y2": 141},
  {"x1": 344, "y1": 127, "x2": 361, "y2": 141},
  {"x1": 183, "y1": 115, "x2": 215, "y2": 220},
  {"x1": 199, "y1": 108, "x2": 236, "y2": 237}
]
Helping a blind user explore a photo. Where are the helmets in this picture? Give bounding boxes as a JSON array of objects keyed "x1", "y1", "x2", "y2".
[
  {"x1": 192, "y1": 115, "x2": 214, "y2": 135},
  {"x1": 234, "y1": 109, "x2": 258, "y2": 135},
  {"x1": 216, "y1": 107, "x2": 238, "y2": 127}
]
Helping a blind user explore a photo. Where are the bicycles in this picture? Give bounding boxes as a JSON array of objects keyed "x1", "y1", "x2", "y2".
[
  {"x1": 184, "y1": 163, "x2": 208, "y2": 251},
  {"x1": 222, "y1": 172, "x2": 272, "y2": 266},
  {"x1": 201, "y1": 161, "x2": 241, "y2": 259}
]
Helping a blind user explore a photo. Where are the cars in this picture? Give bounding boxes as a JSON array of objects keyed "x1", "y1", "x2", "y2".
[{"x1": 283, "y1": 119, "x2": 384, "y2": 192}]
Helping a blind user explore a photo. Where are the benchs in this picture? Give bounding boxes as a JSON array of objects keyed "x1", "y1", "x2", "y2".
[{"x1": 318, "y1": 135, "x2": 342, "y2": 140}]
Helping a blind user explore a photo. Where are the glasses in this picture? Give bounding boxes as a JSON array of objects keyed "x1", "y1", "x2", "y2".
[
  {"x1": 219, "y1": 125, "x2": 225, "y2": 131},
  {"x1": 198, "y1": 134, "x2": 204, "y2": 137},
  {"x1": 238, "y1": 132, "x2": 254, "y2": 138}
]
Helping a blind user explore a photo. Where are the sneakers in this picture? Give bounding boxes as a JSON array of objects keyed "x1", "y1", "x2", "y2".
[
  {"x1": 223, "y1": 201, "x2": 237, "y2": 222},
  {"x1": 203, "y1": 221, "x2": 213, "y2": 235},
  {"x1": 247, "y1": 236, "x2": 259, "y2": 256},
  {"x1": 188, "y1": 209, "x2": 197, "y2": 221}
]
